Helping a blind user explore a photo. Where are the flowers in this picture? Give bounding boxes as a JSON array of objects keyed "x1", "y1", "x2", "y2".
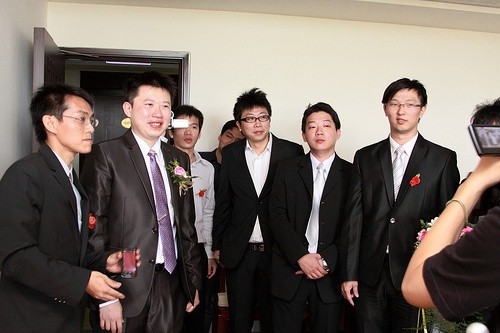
[
  {"x1": 416, "y1": 217, "x2": 496, "y2": 333},
  {"x1": 410, "y1": 174, "x2": 421, "y2": 187},
  {"x1": 89, "y1": 212, "x2": 96, "y2": 229},
  {"x1": 167, "y1": 157, "x2": 203, "y2": 196},
  {"x1": 196, "y1": 188, "x2": 208, "y2": 197}
]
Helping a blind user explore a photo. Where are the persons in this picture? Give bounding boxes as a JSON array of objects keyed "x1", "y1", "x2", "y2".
[
  {"x1": 401, "y1": 96, "x2": 500, "y2": 333},
  {"x1": 0, "y1": 83, "x2": 143, "y2": 333},
  {"x1": 212, "y1": 88, "x2": 304, "y2": 333},
  {"x1": 340, "y1": 78, "x2": 460, "y2": 333},
  {"x1": 167, "y1": 105, "x2": 245, "y2": 333},
  {"x1": 271, "y1": 102, "x2": 352, "y2": 332},
  {"x1": 77, "y1": 70, "x2": 201, "y2": 333}
]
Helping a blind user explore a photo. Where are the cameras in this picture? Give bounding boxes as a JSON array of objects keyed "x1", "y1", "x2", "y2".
[{"x1": 468, "y1": 124, "x2": 500, "y2": 155}]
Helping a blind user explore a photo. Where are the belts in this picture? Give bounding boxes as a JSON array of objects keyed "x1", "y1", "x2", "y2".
[{"x1": 246, "y1": 243, "x2": 265, "y2": 252}]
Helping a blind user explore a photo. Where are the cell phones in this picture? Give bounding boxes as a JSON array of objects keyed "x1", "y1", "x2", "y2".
[{"x1": 120, "y1": 246, "x2": 138, "y2": 278}]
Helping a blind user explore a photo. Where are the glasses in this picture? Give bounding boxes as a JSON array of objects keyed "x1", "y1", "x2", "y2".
[
  {"x1": 58, "y1": 113, "x2": 101, "y2": 129},
  {"x1": 237, "y1": 114, "x2": 270, "y2": 123},
  {"x1": 387, "y1": 101, "x2": 422, "y2": 111}
]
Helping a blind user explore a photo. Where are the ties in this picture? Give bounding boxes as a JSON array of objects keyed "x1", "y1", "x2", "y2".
[
  {"x1": 306, "y1": 163, "x2": 325, "y2": 254},
  {"x1": 146, "y1": 149, "x2": 178, "y2": 274},
  {"x1": 392, "y1": 145, "x2": 406, "y2": 199}
]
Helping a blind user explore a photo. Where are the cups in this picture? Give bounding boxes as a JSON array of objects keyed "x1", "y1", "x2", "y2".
[{"x1": 121, "y1": 249, "x2": 137, "y2": 279}]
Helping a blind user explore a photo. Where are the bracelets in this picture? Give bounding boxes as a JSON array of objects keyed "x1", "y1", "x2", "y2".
[{"x1": 446, "y1": 199, "x2": 468, "y2": 228}]
